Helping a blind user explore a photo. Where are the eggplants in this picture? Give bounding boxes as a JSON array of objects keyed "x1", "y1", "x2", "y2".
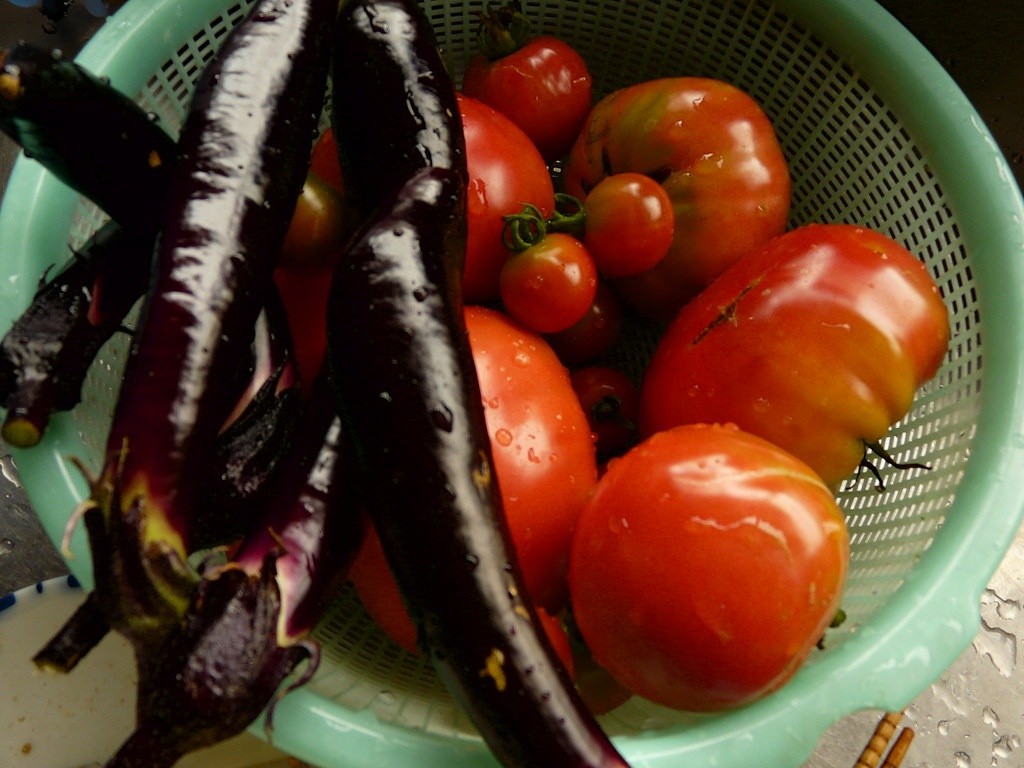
[{"x1": 0, "y1": 0, "x2": 642, "y2": 768}]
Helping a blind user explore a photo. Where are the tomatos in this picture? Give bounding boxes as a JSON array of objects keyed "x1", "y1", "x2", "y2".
[{"x1": 270, "y1": 0, "x2": 951, "y2": 717}]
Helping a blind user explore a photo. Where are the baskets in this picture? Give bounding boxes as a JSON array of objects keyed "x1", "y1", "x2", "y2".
[{"x1": 0, "y1": 0, "x2": 1024, "y2": 768}]
